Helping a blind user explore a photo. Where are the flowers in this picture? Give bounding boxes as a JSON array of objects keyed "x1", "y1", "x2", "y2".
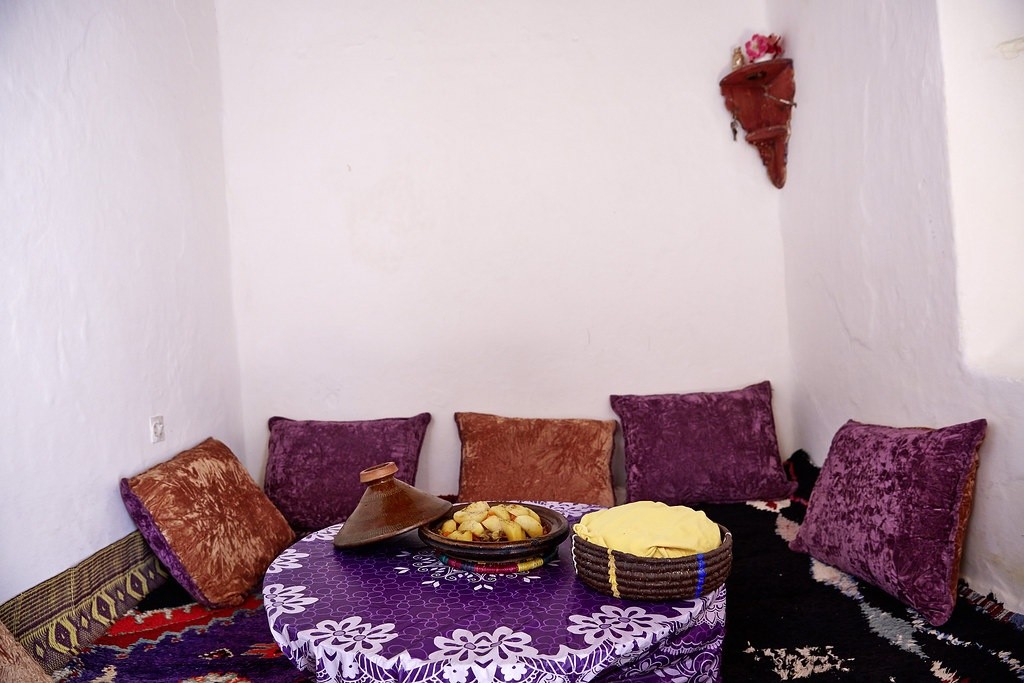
[{"x1": 745, "y1": 32, "x2": 782, "y2": 63}]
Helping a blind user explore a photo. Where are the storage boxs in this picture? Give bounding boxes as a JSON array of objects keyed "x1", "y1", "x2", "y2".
[{"x1": 572, "y1": 508, "x2": 734, "y2": 600}]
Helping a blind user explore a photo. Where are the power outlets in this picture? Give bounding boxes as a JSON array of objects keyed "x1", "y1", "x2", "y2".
[{"x1": 149, "y1": 414, "x2": 167, "y2": 443}]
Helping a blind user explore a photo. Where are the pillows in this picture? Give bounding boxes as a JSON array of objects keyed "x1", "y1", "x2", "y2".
[
  {"x1": 782, "y1": 417, "x2": 988, "y2": 626},
  {"x1": 116, "y1": 436, "x2": 297, "y2": 610},
  {"x1": 608, "y1": 378, "x2": 792, "y2": 508},
  {"x1": 262, "y1": 412, "x2": 433, "y2": 531},
  {"x1": 454, "y1": 412, "x2": 619, "y2": 510}
]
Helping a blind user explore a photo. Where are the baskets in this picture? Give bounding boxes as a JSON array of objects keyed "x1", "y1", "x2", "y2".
[{"x1": 567, "y1": 508, "x2": 733, "y2": 598}]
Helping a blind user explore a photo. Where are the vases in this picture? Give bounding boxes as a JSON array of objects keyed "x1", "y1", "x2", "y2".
[{"x1": 751, "y1": 51, "x2": 776, "y2": 66}]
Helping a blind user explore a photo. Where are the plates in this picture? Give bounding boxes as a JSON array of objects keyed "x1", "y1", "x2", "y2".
[{"x1": 421, "y1": 498, "x2": 571, "y2": 560}]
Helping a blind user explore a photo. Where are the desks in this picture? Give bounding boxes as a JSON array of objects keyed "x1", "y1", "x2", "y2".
[{"x1": 263, "y1": 498, "x2": 729, "y2": 683}]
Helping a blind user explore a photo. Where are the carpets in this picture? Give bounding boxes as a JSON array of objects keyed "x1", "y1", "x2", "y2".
[{"x1": 0, "y1": 447, "x2": 1024, "y2": 683}]
